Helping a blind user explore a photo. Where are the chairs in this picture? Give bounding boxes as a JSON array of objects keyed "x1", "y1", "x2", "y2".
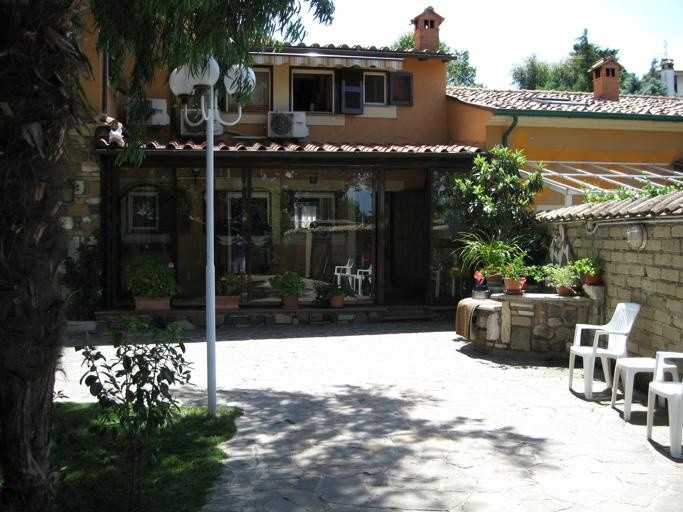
[
  {"x1": 332, "y1": 256, "x2": 373, "y2": 296},
  {"x1": 568, "y1": 301, "x2": 683, "y2": 462},
  {"x1": 429, "y1": 247, "x2": 456, "y2": 298}
]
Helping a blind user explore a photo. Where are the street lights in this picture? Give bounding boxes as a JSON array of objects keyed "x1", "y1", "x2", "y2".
[{"x1": 168, "y1": 47, "x2": 257, "y2": 421}]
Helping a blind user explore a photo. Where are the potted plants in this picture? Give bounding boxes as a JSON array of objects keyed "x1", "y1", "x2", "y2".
[
  {"x1": 448, "y1": 226, "x2": 607, "y2": 296},
  {"x1": 125, "y1": 250, "x2": 184, "y2": 312},
  {"x1": 321, "y1": 285, "x2": 353, "y2": 307},
  {"x1": 270, "y1": 270, "x2": 307, "y2": 308},
  {"x1": 214, "y1": 272, "x2": 249, "y2": 308}
]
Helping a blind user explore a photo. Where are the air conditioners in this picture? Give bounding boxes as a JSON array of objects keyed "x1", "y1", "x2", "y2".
[
  {"x1": 179, "y1": 109, "x2": 221, "y2": 137},
  {"x1": 126, "y1": 97, "x2": 171, "y2": 127},
  {"x1": 266, "y1": 111, "x2": 308, "y2": 139}
]
